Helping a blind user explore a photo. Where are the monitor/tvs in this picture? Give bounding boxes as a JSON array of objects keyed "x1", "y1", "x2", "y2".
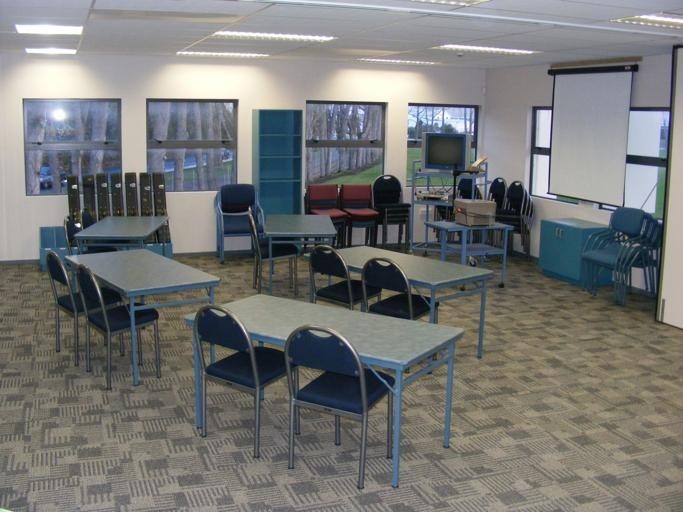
[{"x1": 420, "y1": 132, "x2": 471, "y2": 174}]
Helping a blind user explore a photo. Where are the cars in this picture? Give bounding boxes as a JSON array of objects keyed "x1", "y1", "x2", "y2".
[{"x1": 40, "y1": 165, "x2": 68, "y2": 189}]
